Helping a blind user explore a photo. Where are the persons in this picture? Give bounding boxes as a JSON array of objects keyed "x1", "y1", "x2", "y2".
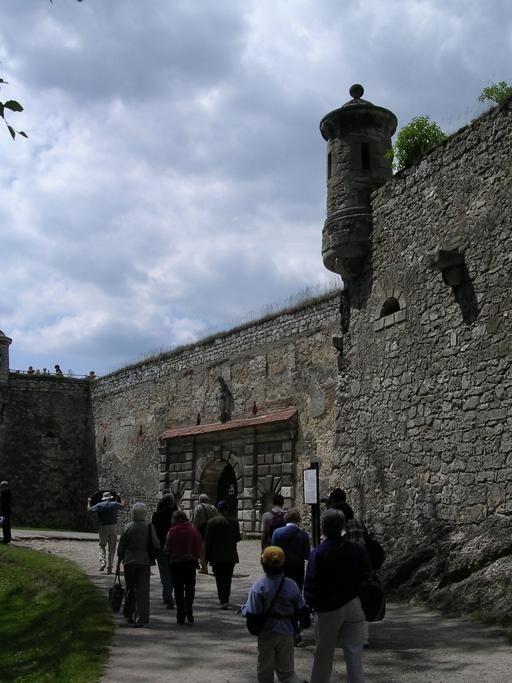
[
  {"x1": 88, "y1": 371, "x2": 97, "y2": 378},
  {"x1": 87, "y1": 490, "x2": 126, "y2": 575},
  {"x1": 1, "y1": 479, "x2": 13, "y2": 544},
  {"x1": 268, "y1": 508, "x2": 312, "y2": 596},
  {"x1": 160, "y1": 509, "x2": 208, "y2": 624},
  {"x1": 189, "y1": 492, "x2": 220, "y2": 573},
  {"x1": 334, "y1": 501, "x2": 368, "y2": 549},
  {"x1": 238, "y1": 544, "x2": 315, "y2": 681},
  {"x1": 55, "y1": 364, "x2": 63, "y2": 375},
  {"x1": 202, "y1": 499, "x2": 242, "y2": 611},
  {"x1": 260, "y1": 493, "x2": 288, "y2": 552},
  {"x1": 115, "y1": 501, "x2": 163, "y2": 626},
  {"x1": 323, "y1": 486, "x2": 347, "y2": 515},
  {"x1": 27, "y1": 366, "x2": 34, "y2": 374},
  {"x1": 150, "y1": 492, "x2": 183, "y2": 609},
  {"x1": 302, "y1": 506, "x2": 378, "y2": 682}
]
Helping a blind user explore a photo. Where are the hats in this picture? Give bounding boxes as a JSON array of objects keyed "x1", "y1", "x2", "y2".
[
  {"x1": 101, "y1": 492, "x2": 114, "y2": 500},
  {"x1": 262, "y1": 546, "x2": 286, "y2": 567},
  {"x1": 273, "y1": 495, "x2": 284, "y2": 505}
]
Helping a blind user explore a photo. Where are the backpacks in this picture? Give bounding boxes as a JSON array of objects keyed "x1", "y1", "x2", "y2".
[{"x1": 367, "y1": 540, "x2": 385, "y2": 570}]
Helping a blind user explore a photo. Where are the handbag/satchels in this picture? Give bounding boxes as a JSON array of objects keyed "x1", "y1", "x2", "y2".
[
  {"x1": 109, "y1": 586, "x2": 124, "y2": 613},
  {"x1": 147, "y1": 541, "x2": 158, "y2": 565},
  {"x1": 247, "y1": 614, "x2": 264, "y2": 635}
]
